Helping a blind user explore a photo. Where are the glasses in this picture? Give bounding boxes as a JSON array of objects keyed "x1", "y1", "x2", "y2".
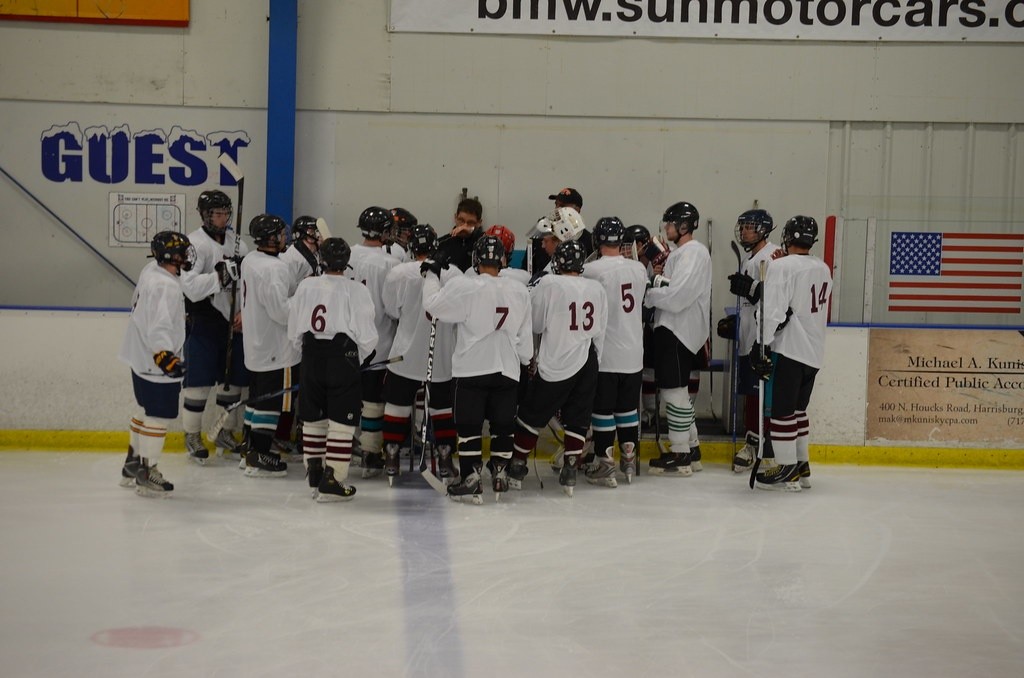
[{"x1": 457, "y1": 217, "x2": 477, "y2": 226}]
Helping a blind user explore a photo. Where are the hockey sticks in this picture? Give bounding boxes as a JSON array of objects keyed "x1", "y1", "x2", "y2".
[
  {"x1": 205, "y1": 354, "x2": 404, "y2": 444},
  {"x1": 217, "y1": 151, "x2": 245, "y2": 392},
  {"x1": 533, "y1": 439, "x2": 545, "y2": 490},
  {"x1": 730, "y1": 241, "x2": 742, "y2": 470},
  {"x1": 706, "y1": 219, "x2": 721, "y2": 425},
  {"x1": 417, "y1": 264, "x2": 449, "y2": 496},
  {"x1": 749, "y1": 259, "x2": 765, "y2": 490},
  {"x1": 654, "y1": 382, "x2": 670, "y2": 458},
  {"x1": 635, "y1": 383, "x2": 642, "y2": 478}
]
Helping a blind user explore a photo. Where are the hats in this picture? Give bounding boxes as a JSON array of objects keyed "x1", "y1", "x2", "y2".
[{"x1": 549, "y1": 187, "x2": 582, "y2": 206}]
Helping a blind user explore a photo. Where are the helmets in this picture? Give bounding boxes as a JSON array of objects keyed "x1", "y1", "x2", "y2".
[
  {"x1": 147, "y1": 231, "x2": 197, "y2": 276},
  {"x1": 196, "y1": 189, "x2": 234, "y2": 234},
  {"x1": 734, "y1": 210, "x2": 818, "y2": 252},
  {"x1": 472, "y1": 203, "x2": 700, "y2": 274},
  {"x1": 249, "y1": 214, "x2": 354, "y2": 274},
  {"x1": 357, "y1": 206, "x2": 438, "y2": 260}
]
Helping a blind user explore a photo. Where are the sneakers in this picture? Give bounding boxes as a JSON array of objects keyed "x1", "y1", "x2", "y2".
[
  {"x1": 120, "y1": 458, "x2": 174, "y2": 498},
  {"x1": 413, "y1": 443, "x2": 635, "y2": 504},
  {"x1": 648, "y1": 447, "x2": 703, "y2": 477},
  {"x1": 641, "y1": 411, "x2": 669, "y2": 435},
  {"x1": 306, "y1": 468, "x2": 356, "y2": 502},
  {"x1": 348, "y1": 451, "x2": 359, "y2": 465},
  {"x1": 186, "y1": 425, "x2": 303, "y2": 478},
  {"x1": 756, "y1": 461, "x2": 811, "y2": 492},
  {"x1": 733, "y1": 430, "x2": 765, "y2": 472},
  {"x1": 361, "y1": 443, "x2": 403, "y2": 486}
]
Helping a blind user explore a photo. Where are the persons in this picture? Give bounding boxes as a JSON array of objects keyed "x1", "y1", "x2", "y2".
[
  {"x1": 756, "y1": 214, "x2": 833, "y2": 491},
  {"x1": 179, "y1": 190, "x2": 248, "y2": 467},
  {"x1": 120, "y1": 231, "x2": 198, "y2": 499},
  {"x1": 240, "y1": 187, "x2": 781, "y2": 504}
]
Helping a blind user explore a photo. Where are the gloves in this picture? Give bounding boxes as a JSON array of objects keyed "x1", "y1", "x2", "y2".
[
  {"x1": 420, "y1": 259, "x2": 441, "y2": 281},
  {"x1": 717, "y1": 315, "x2": 740, "y2": 339},
  {"x1": 752, "y1": 355, "x2": 774, "y2": 381},
  {"x1": 214, "y1": 254, "x2": 244, "y2": 288},
  {"x1": 754, "y1": 306, "x2": 793, "y2": 331},
  {"x1": 153, "y1": 351, "x2": 187, "y2": 377},
  {"x1": 728, "y1": 270, "x2": 760, "y2": 305},
  {"x1": 642, "y1": 303, "x2": 655, "y2": 324},
  {"x1": 646, "y1": 274, "x2": 670, "y2": 290}
]
